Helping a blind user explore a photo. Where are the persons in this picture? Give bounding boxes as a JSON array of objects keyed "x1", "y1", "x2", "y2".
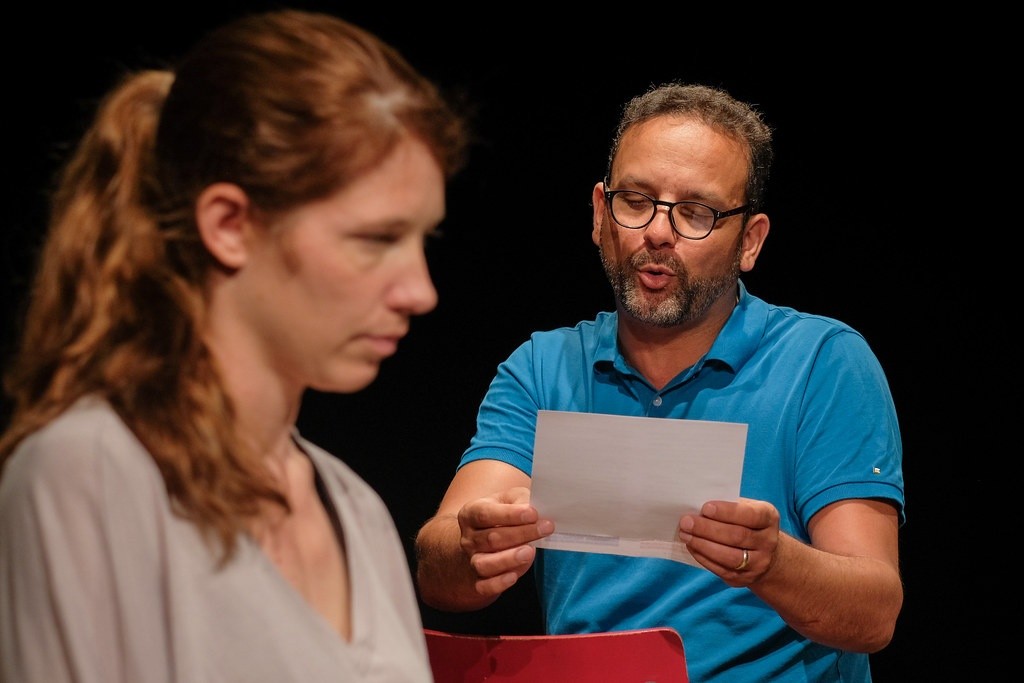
[
  {"x1": 2, "y1": 12, "x2": 438, "y2": 682},
  {"x1": 415, "y1": 84, "x2": 908, "y2": 682}
]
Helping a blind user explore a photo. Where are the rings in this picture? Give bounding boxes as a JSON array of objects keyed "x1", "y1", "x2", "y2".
[{"x1": 735, "y1": 549, "x2": 748, "y2": 571}]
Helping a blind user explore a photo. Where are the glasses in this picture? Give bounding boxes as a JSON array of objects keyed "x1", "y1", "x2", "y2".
[{"x1": 601, "y1": 177, "x2": 748, "y2": 241}]
